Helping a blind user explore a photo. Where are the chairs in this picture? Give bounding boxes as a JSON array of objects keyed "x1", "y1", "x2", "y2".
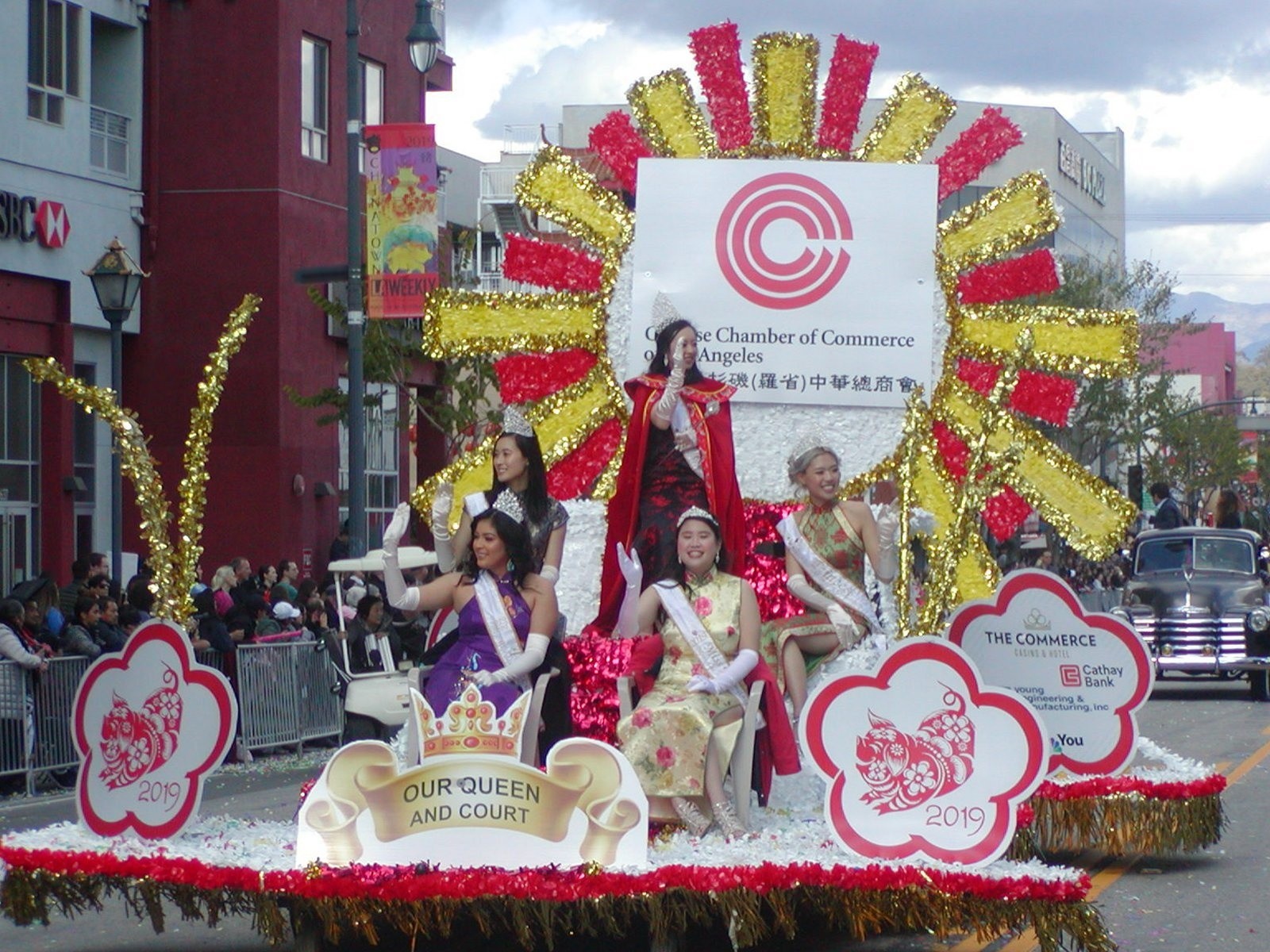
[
  {"x1": 616, "y1": 677, "x2": 766, "y2": 830},
  {"x1": 407, "y1": 665, "x2": 560, "y2": 770}
]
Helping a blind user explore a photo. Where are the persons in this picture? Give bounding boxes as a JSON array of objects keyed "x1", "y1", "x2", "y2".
[
  {"x1": 1139, "y1": 483, "x2": 1185, "y2": 569},
  {"x1": 1214, "y1": 490, "x2": 1242, "y2": 529},
  {"x1": 0, "y1": 553, "x2": 153, "y2": 795},
  {"x1": 613, "y1": 505, "x2": 762, "y2": 842},
  {"x1": 994, "y1": 536, "x2": 1133, "y2": 592},
  {"x1": 191, "y1": 526, "x2": 428, "y2": 673},
  {"x1": 432, "y1": 407, "x2": 570, "y2": 590},
  {"x1": 381, "y1": 501, "x2": 558, "y2": 764},
  {"x1": 597, "y1": 320, "x2": 747, "y2": 631},
  {"x1": 757, "y1": 436, "x2": 902, "y2": 736}
]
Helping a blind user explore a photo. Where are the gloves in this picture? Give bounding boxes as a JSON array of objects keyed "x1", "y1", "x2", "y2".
[
  {"x1": 786, "y1": 574, "x2": 859, "y2": 650},
  {"x1": 431, "y1": 483, "x2": 457, "y2": 574},
  {"x1": 614, "y1": 542, "x2": 643, "y2": 640},
  {"x1": 383, "y1": 502, "x2": 420, "y2": 612},
  {"x1": 877, "y1": 496, "x2": 902, "y2": 580},
  {"x1": 469, "y1": 633, "x2": 549, "y2": 687},
  {"x1": 541, "y1": 565, "x2": 560, "y2": 583},
  {"x1": 687, "y1": 647, "x2": 759, "y2": 695},
  {"x1": 655, "y1": 336, "x2": 686, "y2": 420}
]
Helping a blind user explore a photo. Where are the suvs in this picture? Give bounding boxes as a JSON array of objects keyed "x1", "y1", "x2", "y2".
[{"x1": 1108, "y1": 527, "x2": 1270, "y2": 704}]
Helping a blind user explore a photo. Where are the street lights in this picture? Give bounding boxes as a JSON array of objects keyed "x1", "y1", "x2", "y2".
[
  {"x1": 81, "y1": 234, "x2": 155, "y2": 607},
  {"x1": 340, "y1": 0, "x2": 443, "y2": 554}
]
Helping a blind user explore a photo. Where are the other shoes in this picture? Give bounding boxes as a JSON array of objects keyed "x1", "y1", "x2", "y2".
[
  {"x1": 47, "y1": 766, "x2": 76, "y2": 789},
  {"x1": 311, "y1": 739, "x2": 338, "y2": 751},
  {"x1": 792, "y1": 716, "x2": 802, "y2": 758}
]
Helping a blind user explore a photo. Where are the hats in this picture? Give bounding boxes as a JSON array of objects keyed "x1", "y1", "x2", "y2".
[
  {"x1": 272, "y1": 601, "x2": 300, "y2": 620},
  {"x1": 346, "y1": 586, "x2": 367, "y2": 607}
]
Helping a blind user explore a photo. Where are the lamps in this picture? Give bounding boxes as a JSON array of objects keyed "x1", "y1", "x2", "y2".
[
  {"x1": 63, "y1": 477, "x2": 88, "y2": 491},
  {"x1": 315, "y1": 482, "x2": 336, "y2": 496}
]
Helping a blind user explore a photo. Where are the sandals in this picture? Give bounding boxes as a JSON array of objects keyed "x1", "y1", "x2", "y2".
[
  {"x1": 710, "y1": 803, "x2": 745, "y2": 841},
  {"x1": 675, "y1": 802, "x2": 710, "y2": 839}
]
